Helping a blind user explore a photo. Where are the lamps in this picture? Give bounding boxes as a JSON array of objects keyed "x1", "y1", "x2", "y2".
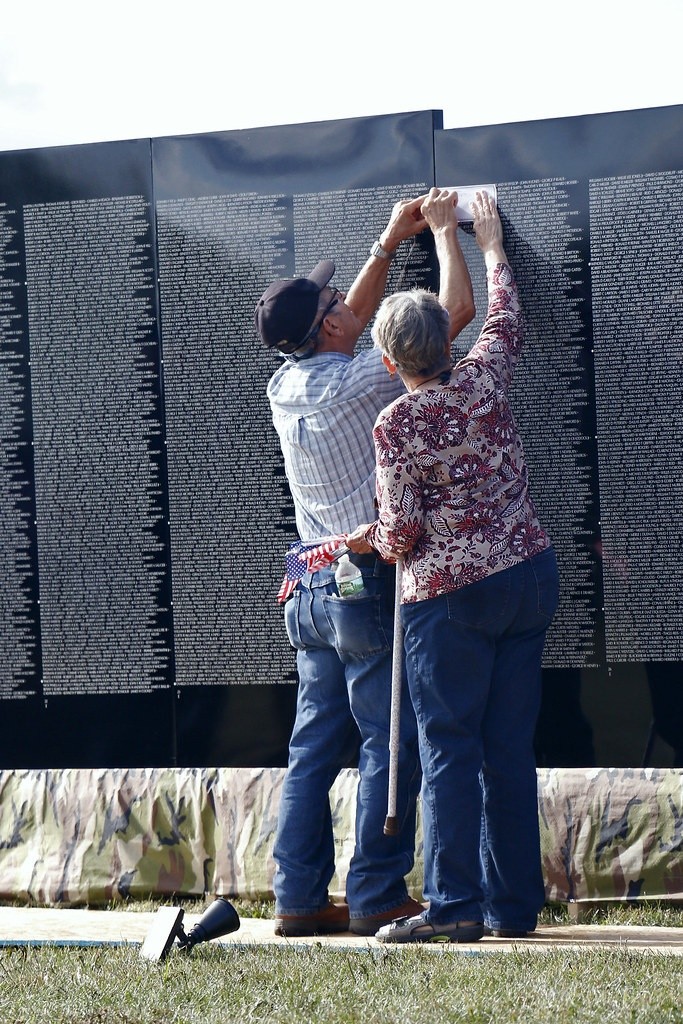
[{"x1": 138, "y1": 898, "x2": 240, "y2": 965}]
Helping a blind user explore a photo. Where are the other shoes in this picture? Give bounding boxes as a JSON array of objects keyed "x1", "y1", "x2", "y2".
[
  {"x1": 350, "y1": 897, "x2": 425, "y2": 932},
  {"x1": 272, "y1": 906, "x2": 350, "y2": 938}
]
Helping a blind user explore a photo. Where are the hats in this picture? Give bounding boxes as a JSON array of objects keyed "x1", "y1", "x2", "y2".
[{"x1": 253, "y1": 260, "x2": 336, "y2": 350}]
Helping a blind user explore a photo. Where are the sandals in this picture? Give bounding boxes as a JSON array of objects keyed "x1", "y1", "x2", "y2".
[{"x1": 371, "y1": 912, "x2": 485, "y2": 941}]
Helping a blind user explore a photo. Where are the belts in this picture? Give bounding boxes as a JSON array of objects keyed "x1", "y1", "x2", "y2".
[{"x1": 331, "y1": 550, "x2": 377, "y2": 568}]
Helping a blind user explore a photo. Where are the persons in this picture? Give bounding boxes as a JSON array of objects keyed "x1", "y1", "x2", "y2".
[
  {"x1": 253, "y1": 186, "x2": 476, "y2": 936},
  {"x1": 343, "y1": 189, "x2": 561, "y2": 945}
]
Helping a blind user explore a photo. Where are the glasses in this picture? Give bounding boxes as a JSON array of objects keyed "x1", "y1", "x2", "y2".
[{"x1": 315, "y1": 286, "x2": 344, "y2": 332}]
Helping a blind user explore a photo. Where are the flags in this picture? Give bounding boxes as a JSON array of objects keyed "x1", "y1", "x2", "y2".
[{"x1": 275, "y1": 532, "x2": 352, "y2": 606}]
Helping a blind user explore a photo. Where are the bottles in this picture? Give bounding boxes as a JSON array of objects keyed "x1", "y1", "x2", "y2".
[{"x1": 334, "y1": 554, "x2": 366, "y2": 600}]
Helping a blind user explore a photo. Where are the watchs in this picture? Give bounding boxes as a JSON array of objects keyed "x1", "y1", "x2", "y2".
[{"x1": 369, "y1": 240, "x2": 397, "y2": 262}]
